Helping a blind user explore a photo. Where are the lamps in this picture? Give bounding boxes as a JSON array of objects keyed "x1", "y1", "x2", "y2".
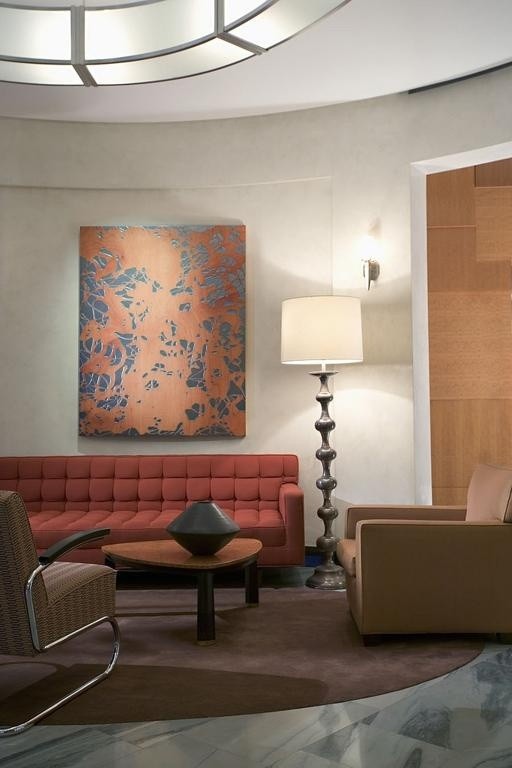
[
  {"x1": 334, "y1": 252, "x2": 380, "y2": 286},
  {"x1": 278, "y1": 293, "x2": 367, "y2": 593}
]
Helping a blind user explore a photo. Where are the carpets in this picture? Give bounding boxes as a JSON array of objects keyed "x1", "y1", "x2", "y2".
[{"x1": 1, "y1": 582, "x2": 489, "y2": 725}]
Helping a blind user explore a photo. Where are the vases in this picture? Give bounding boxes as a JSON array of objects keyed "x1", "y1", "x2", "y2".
[{"x1": 164, "y1": 500, "x2": 243, "y2": 558}]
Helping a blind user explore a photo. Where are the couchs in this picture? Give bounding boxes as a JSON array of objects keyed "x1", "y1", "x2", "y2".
[{"x1": 1, "y1": 454, "x2": 305, "y2": 581}]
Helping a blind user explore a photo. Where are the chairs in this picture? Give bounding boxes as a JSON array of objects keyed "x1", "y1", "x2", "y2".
[
  {"x1": 0, "y1": 490, "x2": 122, "y2": 737},
  {"x1": 335, "y1": 462, "x2": 512, "y2": 649}
]
